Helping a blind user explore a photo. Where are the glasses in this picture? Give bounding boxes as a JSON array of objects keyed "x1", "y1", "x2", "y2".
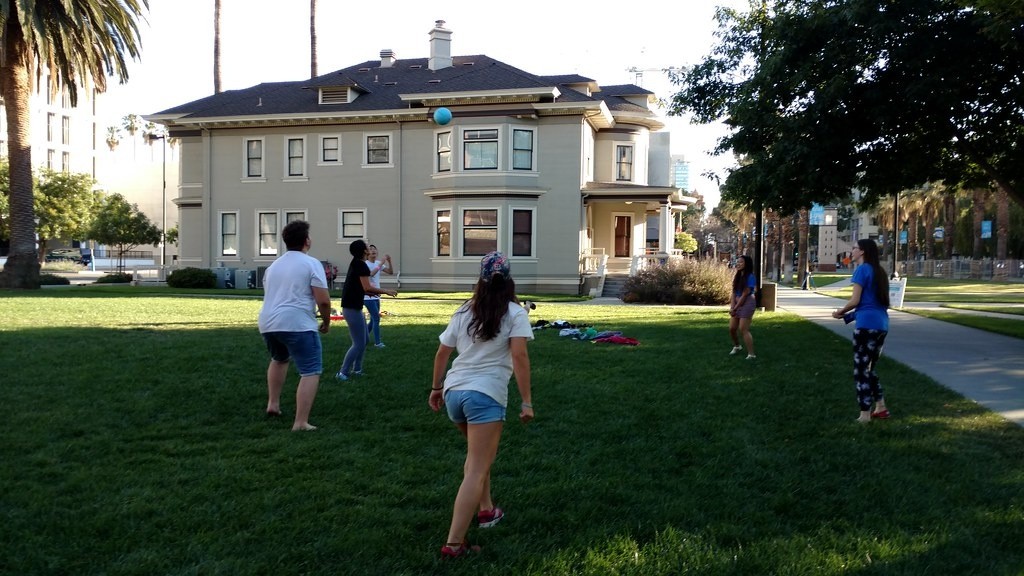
[{"x1": 851, "y1": 246, "x2": 861, "y2": 251}]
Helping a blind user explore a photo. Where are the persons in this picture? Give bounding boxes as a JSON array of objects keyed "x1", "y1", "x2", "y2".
[
  {"x1": 258, "y1": 217, "x2": 331, "y2": 432},
  {"x1": 728, "y1": 255, "x2": 756, "y2": 359},
  {"x1": 832, "y1": 239, "x2": 891, "y2": 425},
  {"x1": 362, "y1": 244, "x2": 394, "y2": 348},
  {"x1": 335, "y1": 239, "x2": 398, "y2": 380},
  {"x1": 429, "y1": 251, "x2": 534, "y2": 556},
  {"x1": 324, "y1": 263, "x2": 339, "y2": 290}
]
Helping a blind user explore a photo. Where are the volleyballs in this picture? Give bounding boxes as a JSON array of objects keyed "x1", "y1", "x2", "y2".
[{"x1": 432, "y1": 106, "x2": 453, "y2": 126}]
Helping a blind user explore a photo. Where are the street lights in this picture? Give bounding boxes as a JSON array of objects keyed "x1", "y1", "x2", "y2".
[{"x1": 148, "y1": 134, "x2": 166, "y2": 268}]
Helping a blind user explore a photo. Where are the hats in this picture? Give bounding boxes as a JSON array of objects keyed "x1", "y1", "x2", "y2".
[{"x1": 480, "y1": 251, "x2": 510, "y2": 282}]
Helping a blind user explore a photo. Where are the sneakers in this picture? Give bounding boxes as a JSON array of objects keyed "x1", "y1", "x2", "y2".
[
  {"x1": 440, "y1": 538, "x2": 481, "y2": 562},
  {"x1": 477, "y1": 504, "x2": 505, "y2": 527}
]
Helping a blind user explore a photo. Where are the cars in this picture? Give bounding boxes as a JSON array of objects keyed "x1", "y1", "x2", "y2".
[{"x1": 45, "y1": 248, "x2": 91, "y2": 266}]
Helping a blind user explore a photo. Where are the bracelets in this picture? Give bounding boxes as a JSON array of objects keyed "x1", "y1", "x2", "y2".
[
  {"x1": 431, "y1": 385, "x2": 444, "y2": 391},
  {"x1": 521, "y1": 402, "x2": 533, "y2": 409}
]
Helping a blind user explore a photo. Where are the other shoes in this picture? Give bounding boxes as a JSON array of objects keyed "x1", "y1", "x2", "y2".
[
  {"x1": 871, "y1": 409, "x2": 891, "y2": 419},
  {"x1": 335, "y1": 372, "x2": 349, "y2": 381},
  {"x1": 352, "y1": 370, "x2": 366, "y2": 375},
  {"x1": 291, "y1": 422, "x2": 317, "y2": 433},
  {"x1": 375, "y1": 341, "x2": 386, "y2": 348},
  {"x1": 267, "y1": 408, "x2": 282, "y2": 418},
  {"x1": 746, "y1": 354, "x2": 757, "y2": 360},
  {"x1": 729, "y1": 345, "x2": 743, "y2": 354}
]
